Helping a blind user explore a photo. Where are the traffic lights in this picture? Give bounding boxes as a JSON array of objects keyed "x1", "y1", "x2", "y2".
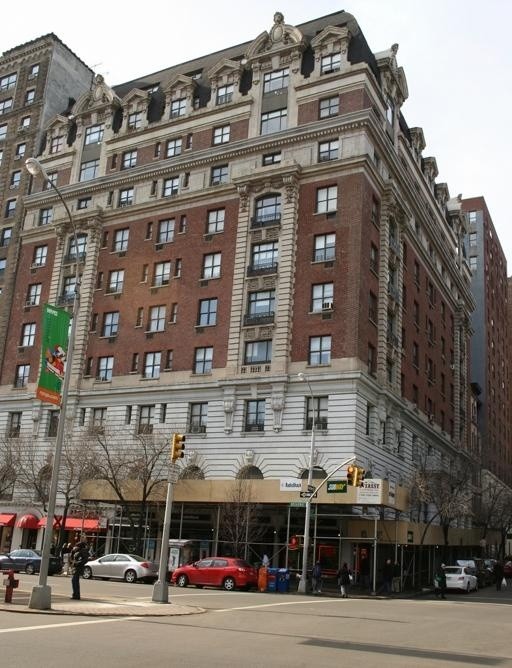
[
  {"x1": 291, "y1": 536, "x2": 301, "y2": 547},
  {"x1": 356, "y1": 467, "x2": 366, "y2": 488},
  {"x1": 346, "y1": 465, "x2": 358, "y2": 487},
  {"x1": 170, "y1": 432, "x2": 186, "y2": 463}
]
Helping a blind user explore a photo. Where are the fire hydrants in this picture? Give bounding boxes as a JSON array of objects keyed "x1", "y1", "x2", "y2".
[{"x1": 1, "y1": 567, "x2": 17, "y2": 603}]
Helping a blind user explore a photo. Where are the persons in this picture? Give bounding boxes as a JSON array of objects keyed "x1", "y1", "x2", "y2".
[
  {"x1": 69, "y1": 542, "x2": 89, "y2": 600},
  {"x1": 51, "y1": 542, "x2": 95, "y2": 577},
  {"x1": 391, "y1": 560, "x2": 401, "y2": 593},
  {"x1": 312, "y1": 560, "x2": 322, "y2": 592},
  {"x1": 504, "y1": 561, "x2": 511, "y2": 592},
  {"x1": 493, "y1": 559, "x2": 504, "y2": 592},
  {"x1": 358, "y1": 554, "x2": 370, "y2": 593},
  {"x1": 383, "y1": 558, "x2": 393, "y2": 595},
  {"x1": 337, "y1": 563, "x2": 352, "y2": 598},
  {"x1": 435, "y1": 563, "x2": 447, "y2": 600}
]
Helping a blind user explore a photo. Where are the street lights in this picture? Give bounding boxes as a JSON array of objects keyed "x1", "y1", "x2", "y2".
[
  {"x1": 296, "y1": 371, "x2": 316, "y2": 593},
  {"x1": 22, "y1": 155, "x2": 82, "y2": 610}
]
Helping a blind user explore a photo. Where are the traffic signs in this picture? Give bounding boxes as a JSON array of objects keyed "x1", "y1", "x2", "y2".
[
  {"x1": 307, "y1": 484, "x2": 316, "y2": 492},
  {"x1": 299, "y1": 492, "x2": 318, "y2": 498},
  {"x1": 166, "y1": 462, "x2": 181, "y2": 484}
]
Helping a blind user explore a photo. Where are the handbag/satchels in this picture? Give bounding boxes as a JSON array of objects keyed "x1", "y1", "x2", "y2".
[{"x1": 348, "y1": 567, "x2": 354, "y2": 581}]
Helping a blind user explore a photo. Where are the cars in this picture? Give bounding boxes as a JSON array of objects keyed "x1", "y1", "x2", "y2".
[
  {"x1": 81, "y1": 551, "x2": 159, "y2": 583},
  {"x1": 433, "y1": 557, "x2": 512, "y2": 594},
  {"x1": 169, "y1": 555, "x2": 259, "y2": 591},
  {"x1": 0, "y1": 546, "x2": 64, "y2": 577}
]
